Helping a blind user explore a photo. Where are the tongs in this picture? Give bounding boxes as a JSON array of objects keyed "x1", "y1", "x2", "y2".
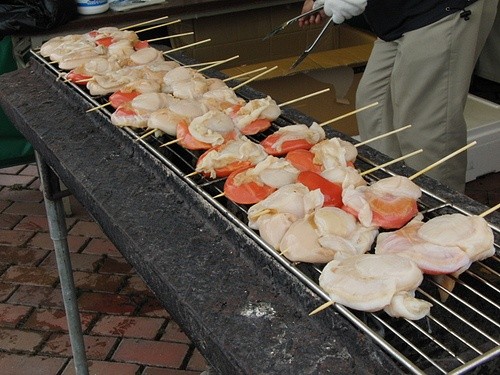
[{"x1": 262, "y1": 5, "x2": 334, "y2": 70}]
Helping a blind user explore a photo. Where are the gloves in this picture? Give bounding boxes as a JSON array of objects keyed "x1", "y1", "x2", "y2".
[{"x1": 312, "y1": 0, "x2": 368, "y2": 25}]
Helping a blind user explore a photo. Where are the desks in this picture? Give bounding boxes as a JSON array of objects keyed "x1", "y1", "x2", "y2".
[{"x1": 28, "y1": 0, "x2": 277, "y2": 50}]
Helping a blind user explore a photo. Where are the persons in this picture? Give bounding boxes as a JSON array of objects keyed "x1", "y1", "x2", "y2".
[{"x1": 293, "y1": 1, "x2": 497, "y2": 195}]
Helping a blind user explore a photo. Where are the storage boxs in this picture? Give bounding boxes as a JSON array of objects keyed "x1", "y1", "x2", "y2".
[
  {"x1": 165, "y1": 1, "x2": 378, "y2": 136},
  {"x1": 463, "y1": 93, "x2": 500, "y2": 182}
]
